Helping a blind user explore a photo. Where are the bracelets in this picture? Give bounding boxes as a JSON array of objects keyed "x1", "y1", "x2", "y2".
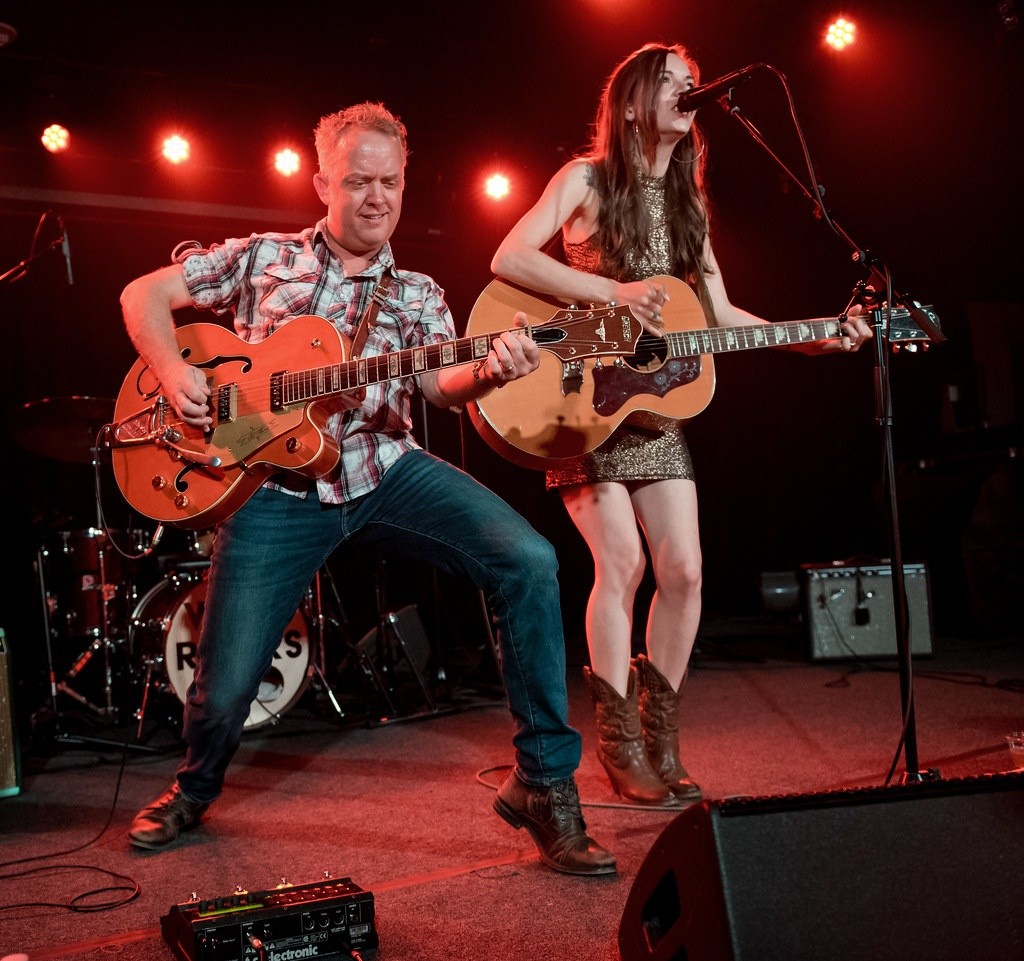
[{"x1": 473, "y1": 359, "x2": 506, "y2": 389}]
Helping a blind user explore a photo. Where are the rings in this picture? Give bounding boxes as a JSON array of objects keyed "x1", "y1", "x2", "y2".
[
  {"x1": 653, "y1": 313, "x2": 658, "y2": 321},
  {"x1": 503, "y1": 364, "x2": 515, "y2": 372},
  {"x1": 849, "y1": 342, "x2": 856, "y2": 346},
  {"x1": 840, "y1": 345, "x2": 849, "y2": 352}
]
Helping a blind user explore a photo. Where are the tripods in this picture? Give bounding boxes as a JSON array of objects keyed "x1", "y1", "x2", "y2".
[{"x1": 21, "y1": 428, "x2": 173, "y2": 761}]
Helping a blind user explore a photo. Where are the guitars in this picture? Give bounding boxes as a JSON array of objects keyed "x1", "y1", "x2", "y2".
[
  {"x1": 465, "y1": 275, "x2": 941, "y2": 470},
  {"x1": 111, "y1": 298, "x2": 644, "y2": 529}
]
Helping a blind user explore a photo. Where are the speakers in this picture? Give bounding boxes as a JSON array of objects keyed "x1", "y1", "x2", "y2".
[
  {"x1": 794, "y1": 561, "x2": 936, "y2": 662},
  {"x1": 335, "y1": 602, "x2": 441, "y2": 714},
  {"x1": 616, "y1": 771, "x2": 1024, "y2": 961}
]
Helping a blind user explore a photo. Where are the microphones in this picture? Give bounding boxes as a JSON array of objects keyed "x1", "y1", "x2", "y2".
[
  {"x1": 854, "y1": 571, "x2": 870, "y2": 625},
  {"x1": 676, "y1": 64, "x2": 766, "y2": 113},
  {"x1": 56, "y1": 214, "x2": 73, "y2": 286}
]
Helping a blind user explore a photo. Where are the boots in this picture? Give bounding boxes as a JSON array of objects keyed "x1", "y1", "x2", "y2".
[
  {"x1": 633, "y1": 654, "x2": 702, "y2": 798},
  {"x1": 582, "y1": 663, "x2": 680, "y2": 806}
]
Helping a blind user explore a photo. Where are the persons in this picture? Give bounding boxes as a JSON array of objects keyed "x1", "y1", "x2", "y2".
[
  {"x1": 491, "y1": 45, "x2": 875, "y2": 805},
  {"x1": 120, "y1": 98, "x2": 619, "y2": 878}
]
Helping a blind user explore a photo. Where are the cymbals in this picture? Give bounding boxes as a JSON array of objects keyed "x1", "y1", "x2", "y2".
[{"x1": 13, "y1": 395, "x2": 116, "y2": 465}]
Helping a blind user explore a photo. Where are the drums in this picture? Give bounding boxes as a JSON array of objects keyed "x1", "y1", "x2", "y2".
[
  {"x1": 58, "y1": 528, "x2": 130, "y2": 637},
  {"x1": 130, "y1": 570, "x2": 318, "y2": 731}
]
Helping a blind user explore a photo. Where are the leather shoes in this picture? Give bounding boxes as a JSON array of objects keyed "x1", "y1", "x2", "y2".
[
  {"x1": 126, "y1": 783, "x2": 208, "y2": 850},
  {"x1": 492, "y1": 766, "x2": 618, "y2": 874}
]
